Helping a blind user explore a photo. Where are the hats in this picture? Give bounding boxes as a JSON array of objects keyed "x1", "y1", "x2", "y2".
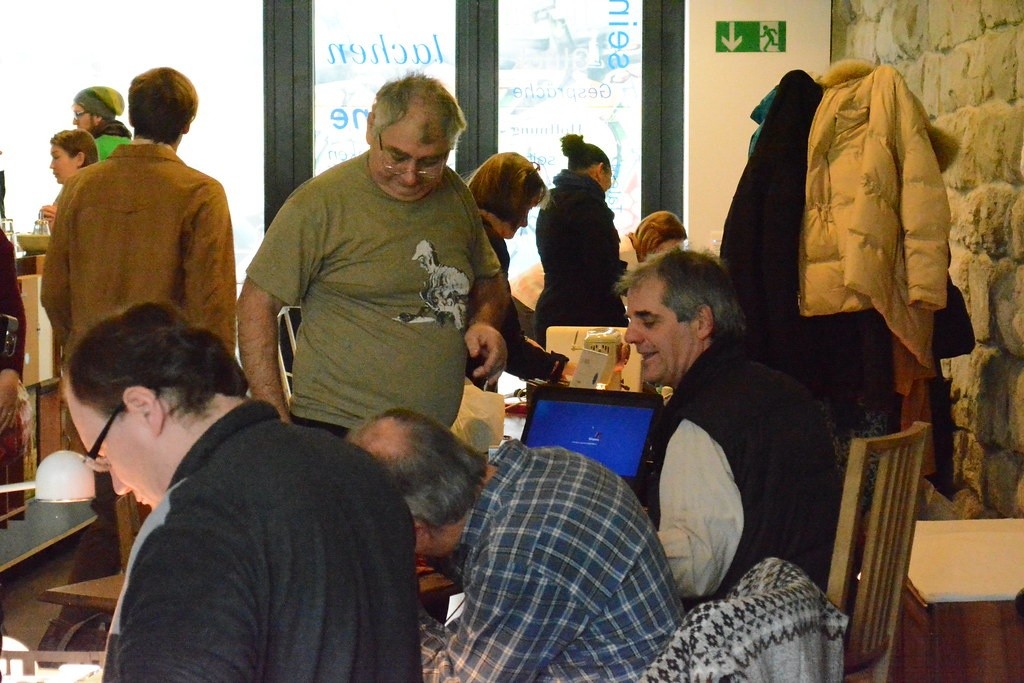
[{"x1": 74, "y1": 86, "x2": 127, "y2": 121}]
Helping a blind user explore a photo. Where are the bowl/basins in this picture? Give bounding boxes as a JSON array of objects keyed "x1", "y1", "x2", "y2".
[{"x1": 16, "y1": 233, "x2": 52, "y2": 255}]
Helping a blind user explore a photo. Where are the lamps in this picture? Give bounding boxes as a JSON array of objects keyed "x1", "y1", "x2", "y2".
[{"x1": 0, "y1": 450, "x2": 97, "y2": 504}]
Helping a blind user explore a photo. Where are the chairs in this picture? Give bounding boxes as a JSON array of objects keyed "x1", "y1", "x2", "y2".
[
  {"x1": 37, "y1": 487, "x2": 153, "y2": 614},
  {"x1": 822, "y1": 419, "x2": 930, "y2": 683}
]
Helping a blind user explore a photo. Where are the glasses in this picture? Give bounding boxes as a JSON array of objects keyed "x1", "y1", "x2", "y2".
[
  {"x1": 375, "y1": 127, "x2": 450, "y2": 187},
  {"x1": 79, "y1": 401, "x2": 146, "y2": 476},
  {"x1": 599, "y1": 162, "x2": 617, "y2": 185},
  {"x1": 73, "y1": 109, "x2": 95, "y2": 120}
]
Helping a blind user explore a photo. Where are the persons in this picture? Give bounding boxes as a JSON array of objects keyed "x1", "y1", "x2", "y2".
[
  {"x1": 0, "y1": 69, "x2": 236, "y2": 527},
  {"x1": 459, "y1": 133, "x2": 689, "y2": 393},
  {"x1": 613, "y1": 249, "x2": 846, "y2": 613},
  {"x1": 343, "y1": 409, "x2": 691, "y2": 682},
  {"x1": 233, "y1": 74, "x2": 513, "y2": 437},
  {"x1": 63, "y1": 298, "x2": 426, "y2": 682}
]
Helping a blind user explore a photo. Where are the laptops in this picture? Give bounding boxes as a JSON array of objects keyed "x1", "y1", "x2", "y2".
[{"x1": 520, "y1": 386, "x2": 663, "y2": 507}]
